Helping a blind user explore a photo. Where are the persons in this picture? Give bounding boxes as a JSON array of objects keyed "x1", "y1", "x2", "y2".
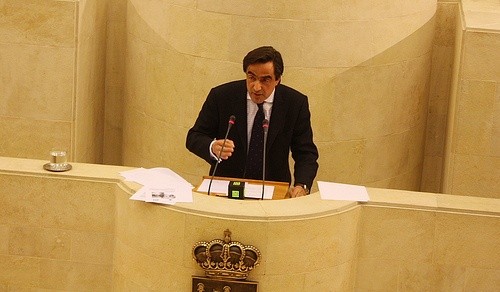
[{"x1": 185, "y1": 46, "x2": 319, "y2": 198}]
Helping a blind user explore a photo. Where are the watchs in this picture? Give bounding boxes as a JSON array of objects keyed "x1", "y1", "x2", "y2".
[{"x1": 295, "y1": 183, "x2": 310, "y2": 195}]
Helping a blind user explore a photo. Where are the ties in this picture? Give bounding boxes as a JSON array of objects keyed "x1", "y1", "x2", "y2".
[{"x1": 244, "y1": 102, "x2": 265, "y2": 180}]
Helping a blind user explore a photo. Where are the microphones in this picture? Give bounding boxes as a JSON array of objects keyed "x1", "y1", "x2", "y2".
[
  {"x1": 260, "y1": 119, "x2": 269, "y2": 200},
  {"x1": 208, "y1": 115, "x2": 236, "y2": 195}
]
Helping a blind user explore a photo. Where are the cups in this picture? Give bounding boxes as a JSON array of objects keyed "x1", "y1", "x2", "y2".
[{"x1": 50, "y1": 148, "x2": 67, "y2": 169}]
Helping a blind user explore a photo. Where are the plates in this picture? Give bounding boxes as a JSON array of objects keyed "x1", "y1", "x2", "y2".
[{"x1": 43, "y1": 163, "x2": 72, "y2": 171}]
